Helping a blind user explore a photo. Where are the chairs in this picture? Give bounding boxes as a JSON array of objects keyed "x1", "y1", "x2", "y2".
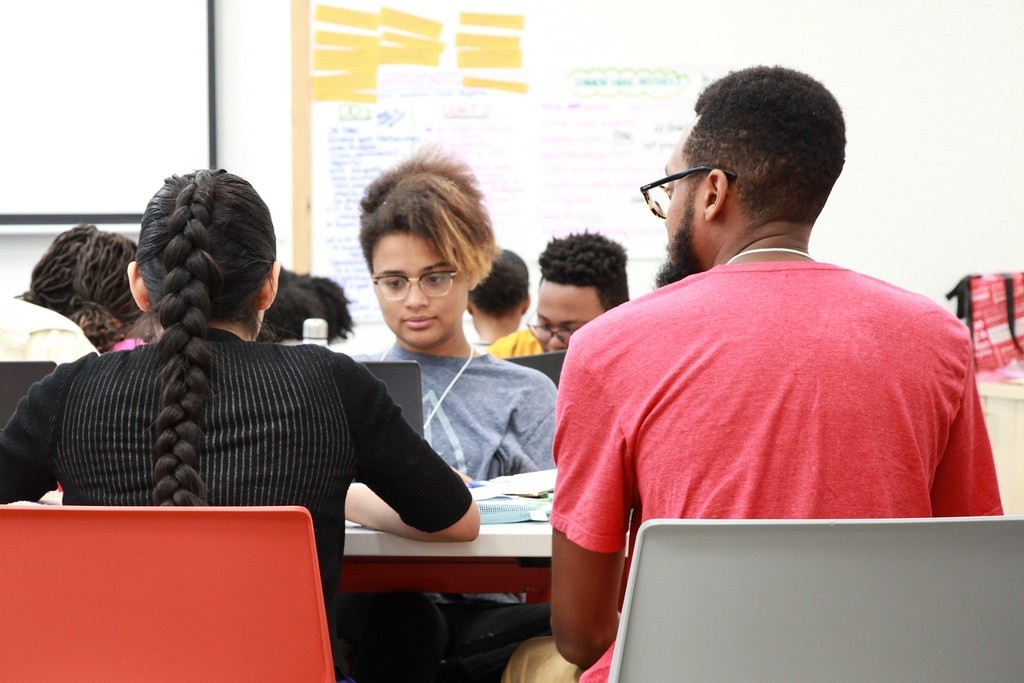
[
  {"x1": 0, "y1": 505, "x2": 335, "y2": 683},
  {"x1": 605, "y1": 515, "x2": 1024, "y2": 683}
]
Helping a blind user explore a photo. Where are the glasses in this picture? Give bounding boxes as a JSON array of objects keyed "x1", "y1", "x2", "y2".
[
  {"x1": 640, "y1": 166, "x2": 736, "y2": 219},
  {"x1": 370, "y1": 267, "x2": 460, "y2": 301},
  {"x1": 526, "y1": 310, "x2": 575, "y2": 346}
]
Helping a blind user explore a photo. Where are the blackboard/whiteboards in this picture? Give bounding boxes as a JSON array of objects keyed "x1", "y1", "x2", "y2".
[{"x1": 0, "y1": 0, "x2": 218, "y2": 237}]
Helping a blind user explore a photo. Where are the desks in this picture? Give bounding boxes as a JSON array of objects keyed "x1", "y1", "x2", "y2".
[{"x1": 337, "y1": 490, "x2": 633, "y2": 599}]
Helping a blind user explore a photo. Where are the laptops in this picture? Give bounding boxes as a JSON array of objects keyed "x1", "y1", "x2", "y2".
[
  {"x1": 504, "y1": 350, "x2": 569, "y2": 390},
  {"x1": 353, "y1": 360, "x2": 425, "y2": 442}
]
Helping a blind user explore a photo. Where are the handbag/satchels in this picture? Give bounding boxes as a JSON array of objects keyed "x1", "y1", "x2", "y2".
[{"x1": 946, "y1": 271, "x2": 1024, "y2": 380}]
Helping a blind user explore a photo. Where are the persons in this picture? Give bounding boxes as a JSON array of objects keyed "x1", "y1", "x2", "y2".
[
  {"x1": 0, "y1": 149, "x2": 630, "y2": 683},
  {"x1": 497, "y1": 66, "x2": 1008, "y2": 683}
]
